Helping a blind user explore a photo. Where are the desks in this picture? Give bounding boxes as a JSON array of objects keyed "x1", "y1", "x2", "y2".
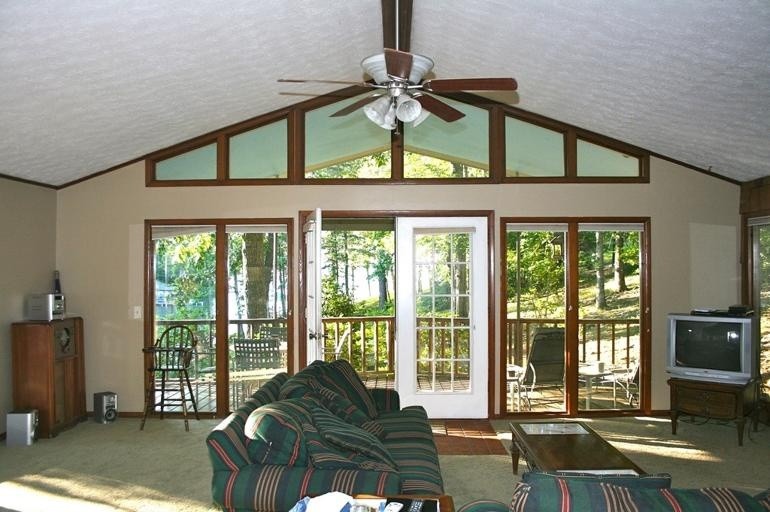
[
  {"x1": 578, "y1": 367, "x2": 617, "y2": 411},
  {"x1": 229, "y1": 341, "x2": 287, "y2": 394}
]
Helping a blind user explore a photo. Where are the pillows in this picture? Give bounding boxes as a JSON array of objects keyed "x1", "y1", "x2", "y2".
[
  {"x1": 522, "y1": 471, "x2": 672, "y2": 489},
  {"x1": 296, "y1": 359, "x2": 395, "y2": 473}
]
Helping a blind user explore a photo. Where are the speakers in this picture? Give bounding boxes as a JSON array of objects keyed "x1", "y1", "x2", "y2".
[
  {"x1": 94, "y1": 392, "x2": 118, "y2": 425},
  {"x1": 5, "y1": 408, "x2": 40, "y2": 449}
]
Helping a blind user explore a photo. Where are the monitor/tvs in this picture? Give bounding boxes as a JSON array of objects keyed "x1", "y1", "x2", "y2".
[{"x1": 664, "y1": 312, "x2": 760, "y2": 385}]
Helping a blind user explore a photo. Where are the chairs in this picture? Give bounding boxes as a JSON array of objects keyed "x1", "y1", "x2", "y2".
[
  {"x1": 234, "y1": 326, "x2": 288, "y2": 408},
  {"x1": 506, "y1": 326, "x2": 564, "y2": 412},
  {"x1": 139, "y1": 324, "x2": 200, "y2": 434}
]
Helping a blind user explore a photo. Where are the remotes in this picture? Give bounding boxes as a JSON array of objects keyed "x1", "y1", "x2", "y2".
[
  {"x1": 383, "y1": 502, "x2": 404, "y2": 512},
  {"x1": 407, "y1": 499, "x2": 425, "y2": 512}
]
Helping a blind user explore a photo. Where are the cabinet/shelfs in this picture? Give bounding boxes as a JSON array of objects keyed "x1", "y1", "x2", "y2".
[
  {"x1": 11, "y1": 317, "x2": 88, "y2": 439},
  {"x1": 668, "y1": 379, "x2": 761, "y2": 447}
]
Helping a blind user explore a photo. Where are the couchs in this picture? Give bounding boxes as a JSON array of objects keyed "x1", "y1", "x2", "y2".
[{"x1": 206, "y1": 373, "x2": 445, "y2": 512}]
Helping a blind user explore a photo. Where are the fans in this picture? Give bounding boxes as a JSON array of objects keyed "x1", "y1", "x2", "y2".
[{"x1": 276, "y1": 1, "x2": 519, "y2": 124}]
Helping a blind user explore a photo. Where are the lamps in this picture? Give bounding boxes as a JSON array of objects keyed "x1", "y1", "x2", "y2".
[{"x1": 356, "y1": 50, "x2": 434, "y2": 130}]
